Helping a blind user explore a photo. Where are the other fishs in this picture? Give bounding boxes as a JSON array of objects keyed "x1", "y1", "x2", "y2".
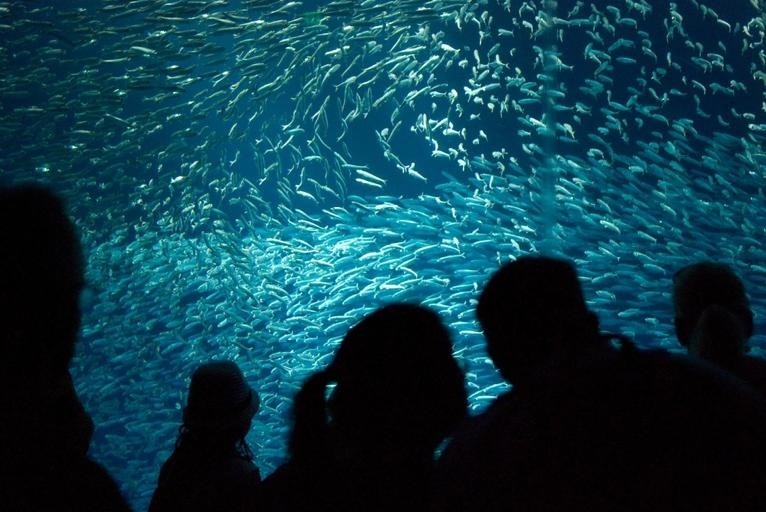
[{"x1": 2, "y1": 0, "x2": 766, "y2": 512}]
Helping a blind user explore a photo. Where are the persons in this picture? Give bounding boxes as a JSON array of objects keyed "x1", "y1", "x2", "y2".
[
  {"x1": 1, "y1": 180, "x2": 130, "y2": 509},
  {"x1": 671, "y1": 258, "x2": 764, "y2": 379},
  {"x1": 262, "y1": 302, "x2": 470, "y2": 511},
  {"x1": 146, "y1": 361, "x2": 262, "y2": 511},
  {"x1": 438, "y1": 255, "x2": 766, "y2": 511}
]
